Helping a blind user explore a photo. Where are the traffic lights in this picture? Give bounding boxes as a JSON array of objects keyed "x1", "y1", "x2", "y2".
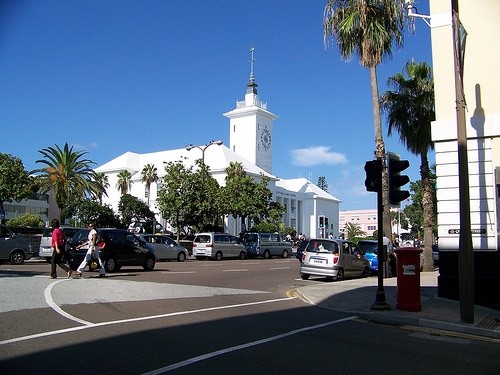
[
  {"x1": 364, "y1": 160, "x2": 380, "y2": 192},
  {"x1": 324, "y1": 217, "x2": 328, "y2": 226},
  {"x1": 386, "y1": 153, "x2": 410, "y2": 208},
  {"x1": 318, "y1": 215, "x2": 325, "y2": 229}
]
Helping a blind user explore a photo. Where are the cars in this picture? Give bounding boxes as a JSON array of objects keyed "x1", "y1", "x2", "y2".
[
  {"x1": 295, "y1": 239, "x2": 308, "y2": 263},
  {"x1": 352, "y1": 239, "x2": 379, "y2": 275},
  {"x1": 299, "y1": 238, "x2": 370, "y2": 282},
  {"x1": 392, "y1": 233, "x2": 422, "y2": 248},
  {"x1": 128, "y1": 233, "x2": 189, "y2": 262}
]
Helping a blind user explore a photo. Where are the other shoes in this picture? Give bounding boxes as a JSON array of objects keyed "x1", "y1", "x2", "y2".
[
  {"x1": 67, "y1": 270, "x2": 73, "y2": 279},
  {"x1": 74, "y1": 272, "x2": 82, "y2": 277},
  {"x1": 49, "y1": 274, "x2": 57, "y2": 279},
  {"x1": 99, "y1": 274, "x2": 105, "y2": 277}
]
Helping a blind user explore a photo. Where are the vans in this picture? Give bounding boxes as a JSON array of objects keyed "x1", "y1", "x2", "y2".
[
  {"x1": 241, "y1": 232, "x2": 292, "y2": 259},
  {"x1": 38, "y1": 226, "x2": 81, "y2": 261},
  {"x1": 0, "y1": 223, "x2": 34, "y2": 266}
]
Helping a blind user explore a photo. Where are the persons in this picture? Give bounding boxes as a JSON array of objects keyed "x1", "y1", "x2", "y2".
[
  {"x1": 50, "y1": 219, "x2": 73, "y2": 279},
  {"x1": 415, "y1": 238, "x2": 424, "y2": 248},
  {"x1": 388, "y1": 240, "x2": 398, "y2": 251},
  {"x1": 75, "y1": 223, "x2": 104, "y2": 277},
  {"x1": 285, "y1": 233, "x2": 308, "y2": 255},
  {"x1": 326, "y1": 233, "x2": 333, "y2": 239}
]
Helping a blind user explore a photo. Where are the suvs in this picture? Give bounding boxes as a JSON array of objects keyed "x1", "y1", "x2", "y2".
[
  {"x1": 66, "y1": 226, "x2": 156, "y2": 274},
  {"x1": 191, "y1": 231, "x2": 235, "y2": 254}
]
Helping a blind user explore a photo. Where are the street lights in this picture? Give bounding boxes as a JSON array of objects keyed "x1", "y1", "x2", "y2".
[{"x1": 185, "y1": 140, "x2": 224, "y2": 165}]
